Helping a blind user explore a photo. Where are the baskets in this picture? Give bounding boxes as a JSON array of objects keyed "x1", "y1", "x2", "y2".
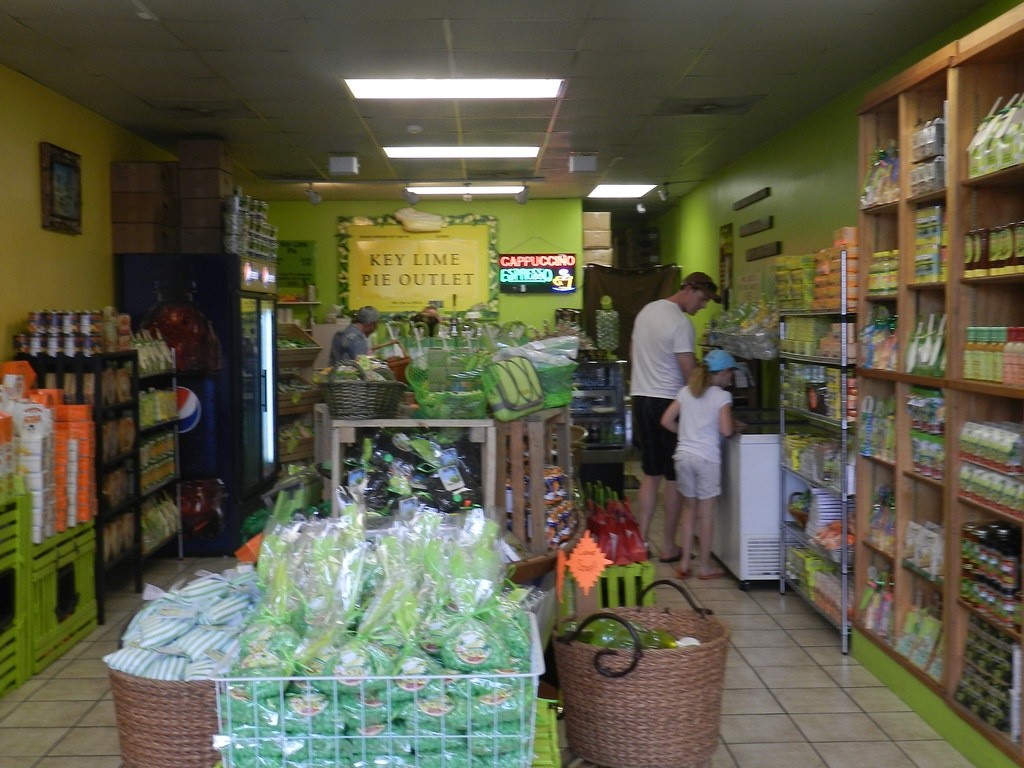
[
  {"x1": 329, "y1": 333, "x2": 576, "y2": 421},
  {"x1": 553, "y1": 579, "x2": 732, "y2": 768},
  {"x1": 109, "y1": 612, "x2": 223, "y2": 768}
]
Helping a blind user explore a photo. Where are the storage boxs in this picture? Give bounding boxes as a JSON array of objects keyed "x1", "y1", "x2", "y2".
[
  {"x1": 109, "y1": 139, "x2": 235, "y2": 254},
  {"x1": 582, "y1": 212, "x2": 614, "y2": 267},
  {"x1": 0, "y1": 358, "x2": 98, "y2": 545}
]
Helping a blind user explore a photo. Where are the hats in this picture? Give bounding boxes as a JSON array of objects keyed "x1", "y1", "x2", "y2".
[
  {"x1": 679, "y1": 271, "x2": 725, "y2": 304},
  {"x1": 705, "y1": 350, "x2": 741, "y2": 372}
]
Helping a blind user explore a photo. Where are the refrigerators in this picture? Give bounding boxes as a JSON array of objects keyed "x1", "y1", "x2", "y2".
[
  {"x1": 693, "y1": 424, "x2": 797, "y2": 585},
  {"x1": 112, "y1": 251, "x2": 278, "y2": 503}
]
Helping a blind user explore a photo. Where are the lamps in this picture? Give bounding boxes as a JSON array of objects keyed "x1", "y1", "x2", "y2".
[
  {"x1": 304, "y1": 181, "x2": 322, "y2": 206},
  {"x1": 513, "y1": 181, "x2": 531, "y2": 204},
  {"x1": 658, "y1": 181, "x2": 670, "y2": 202},
  {"x1": 400, "y1": 181, "x2": 419, "y2": 206}
]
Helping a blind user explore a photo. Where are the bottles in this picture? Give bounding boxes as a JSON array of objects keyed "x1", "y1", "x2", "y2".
[
  {"x1": 962, "y1": 325, "x2": 1024, "y2": 389},
  {"x1": 866, "y1": 250, "x2": 899, "y2": 296},
  {"x1": 963, "y1": 220, "x2": 1024, "y2": 278},
  {"x1": 10, "y1": 310, "x2": 106, "y2": 357},
  {"x1": 957, "y1": 520, "x2": 1024, "y2": 630}
]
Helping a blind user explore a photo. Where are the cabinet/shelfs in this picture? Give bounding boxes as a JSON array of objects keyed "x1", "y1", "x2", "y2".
[
  {"x1": 776, "y1": 0, "x2": 1024, "y2": 767},
  {"x1": 13, "y1": 351, "x2": 144, "y2": 627},
  {"x1": 137, "y1": 346, "x2": 187, "y2": 563},
  {"x1": 272, "y1": 301, "x2": 324, "y2": 469}
]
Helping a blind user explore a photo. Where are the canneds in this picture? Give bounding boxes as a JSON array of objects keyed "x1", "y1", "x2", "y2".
[
  {"x1": 14, "y1": 310, "x2": 104, "y2": 357},
  {"x1": 221, "y1": 193, "x2": 278, "y2": 262}
]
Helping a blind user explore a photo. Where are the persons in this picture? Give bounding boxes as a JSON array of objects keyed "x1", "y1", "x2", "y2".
[
  {"x1": 661, "y1": 349, "x2": 745, "y2": 579},
  {"x1": 423, "y1": 305, "x2": 437, "y2": 317},
  {"x1": 330, "y1": 306, "x2": 378, "y2": 365},
  {"x1": 630, "y1": 273, "x2": 723, "y2": 562}
]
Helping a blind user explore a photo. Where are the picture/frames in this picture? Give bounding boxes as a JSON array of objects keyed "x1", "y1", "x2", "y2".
[{"x1": 40, "y1": 141, "x2": 82, "y2": 236}]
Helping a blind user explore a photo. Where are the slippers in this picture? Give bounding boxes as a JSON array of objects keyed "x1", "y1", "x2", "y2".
[
  {"x1": 658, "y1": 546, "x2": 695, "y2": 564},
  {"x1": 672, "y1": 563, "x2": 689, "y2": 575},
  {"x1": 698, "y1": 566, "x2": 726, "y2": 578}
]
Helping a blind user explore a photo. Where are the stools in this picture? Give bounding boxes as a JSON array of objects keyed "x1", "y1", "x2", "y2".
[{"x1": 595, "y1": 557, "x2": 653, "y2": 609}]
